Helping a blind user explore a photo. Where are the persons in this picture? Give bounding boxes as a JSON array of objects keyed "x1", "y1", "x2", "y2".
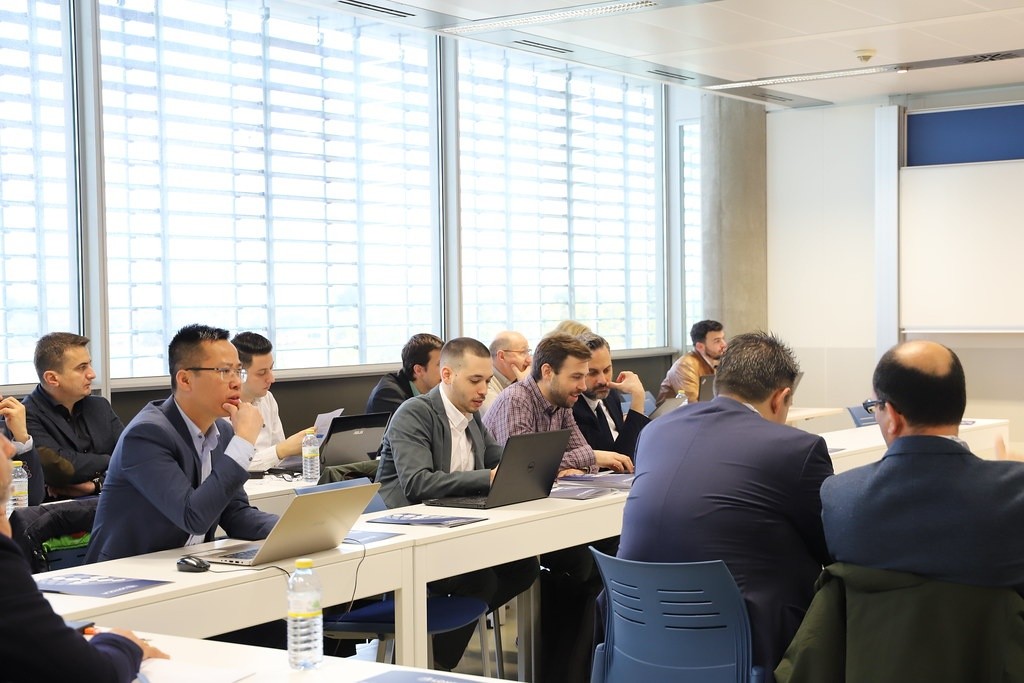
[
  {"x1": 222, "y1": 332, "x2": 318, "y2": 472},
  {"x1": 615, "y1": 331, "x2": 834, "y2": 683},
  {"x1": 571, "y1": 333, "x2": 653, "y2": 472},
  {"x1": 373, "y1": 337, "x2": 504, "y2": 672},
  {"x1": 0, "y1": 432, "x2": 170, "y2": 683},
  {"x1": 365, "y1": 334, "x2": 446, "y2": 416},
  {"x1": 655, "y1": 320, "x2": 726, "y2": 408},
  {"x1": 20, "y1": 332, "x2": 125, "y2": 504},
  {"x1": 0, "y1": 395, "x2": 45, "y2": 507},
  {"x1": 557, "y1": 321, "x2": 591, "y2": 338},
  {"x1": 81, "y1": 325, "x2": 280, "y2": 565},
  {"x1": 481, "y1": 333, "x2": 635, "y2": 648},
  {"x1": 819, "y1": 341, "x2": 1024, "y2": 589},
  {"x1": 479, "y1": 330, "x2": 534, "y2": 418}
]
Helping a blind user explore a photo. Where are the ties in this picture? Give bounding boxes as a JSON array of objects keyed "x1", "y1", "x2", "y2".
[{"x1": 595, "y1": 402, "x2": 614, "y2": 443}]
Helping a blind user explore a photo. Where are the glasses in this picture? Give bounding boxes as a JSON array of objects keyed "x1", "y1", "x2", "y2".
[
  {"x1": 495, "y1": 349, "x2": 531, "y2": 358},
  {"x1": 863, "y1": 398, "x2": 900, "y2": 415},
  {"x1": 184, "y1": 367, "x2": 248, "y2": 384}
]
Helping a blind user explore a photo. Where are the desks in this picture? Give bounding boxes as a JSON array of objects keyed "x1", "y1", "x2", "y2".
[{"x1": 30, "y1": 408, "x2": 1011, "y2": 683}]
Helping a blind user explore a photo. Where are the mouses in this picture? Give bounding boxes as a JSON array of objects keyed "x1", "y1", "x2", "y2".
[{"x1": 177, "y1": 557, "x2": 210, "y2": 572}]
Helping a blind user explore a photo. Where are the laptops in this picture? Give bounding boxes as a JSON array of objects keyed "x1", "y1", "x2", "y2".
[
  {"x1": 648, "y1": 395, "x2": 690, "y2": 421},
  {"x1": 699, "y1": 374, "x2": 716, "y2": 402},
  {"x1": 319, "y1": 412, "x2": 392, "y2": 474},
  {"x1": 421, "y1": 427, "x2": 574, "y2": 509},
  {"x1": 182, "y1": 482, "x2": 382, "y2": 566}
]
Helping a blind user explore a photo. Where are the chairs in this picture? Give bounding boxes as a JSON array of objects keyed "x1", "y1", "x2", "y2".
[{"x1": 289, "y1": 390, "x2": 768, "y2": 683}]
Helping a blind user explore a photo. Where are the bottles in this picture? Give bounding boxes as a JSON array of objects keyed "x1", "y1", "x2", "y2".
[
  {"x1": 5, "y1": 461, "x2": 28, "y2": 521},
  {"x1": 288, "y1": 559, "x2": 324, "y2": 670},
  {"x1": 675, "y1": 390, "x2": 688, "y2": 408},
  {"x1": 302, "y1": 430, "x2": 320, "y2": 483},
  {"x1": 316, "y1": 433, "x2": 326, "y2": 448}
]
